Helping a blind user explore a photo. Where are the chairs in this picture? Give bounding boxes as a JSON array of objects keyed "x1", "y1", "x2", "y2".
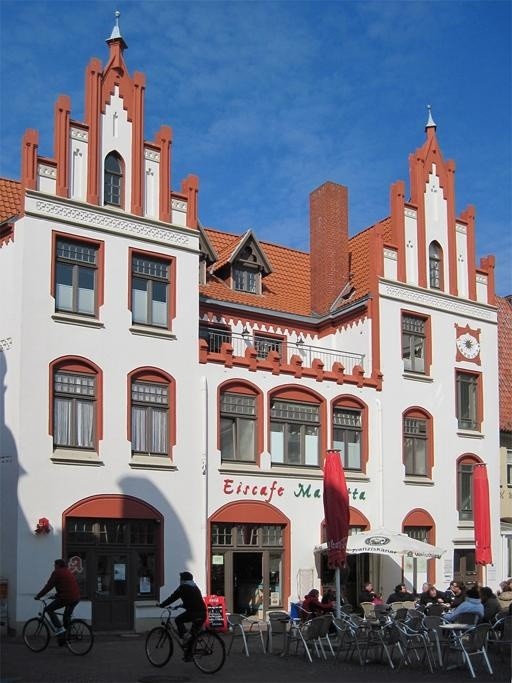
[{"x1": 222, "y1": 589, "x2": 511, "y2": 680}]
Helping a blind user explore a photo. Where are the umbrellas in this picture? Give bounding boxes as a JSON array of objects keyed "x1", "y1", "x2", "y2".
[
  {"x1": 471, "y1": 462, "x2": 492, "y2": 587},
  {"x1": 323, "y1": 448, "x2": 349, "y2": 621}
]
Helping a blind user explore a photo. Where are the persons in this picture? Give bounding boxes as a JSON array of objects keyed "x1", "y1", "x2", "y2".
[
  {"x1": 159, "y1": 572, "x2": 207, "y2": 662},
  {"x1": 299, "y1": 578, "x2": 511, "y2": 647},
  {"x1": 34, "y1": 558, "x2": 80, "y2": 648}
]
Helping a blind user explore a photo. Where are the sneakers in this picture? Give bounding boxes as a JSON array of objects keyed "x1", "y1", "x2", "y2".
[
  {"x1": 55, "y1": 625, "x2": 67, "y2": 637},
  {"x1": 182, "y1": 632, "x2": 192, "y2": 646}
]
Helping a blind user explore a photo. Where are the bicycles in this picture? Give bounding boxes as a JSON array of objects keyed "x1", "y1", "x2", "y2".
[
  {"x1": 143, "y1": 600, "x2": 227, "y2": 674},
  {"x1": 22, "y1": 595, "x2": 93, "y2": 655}
]
